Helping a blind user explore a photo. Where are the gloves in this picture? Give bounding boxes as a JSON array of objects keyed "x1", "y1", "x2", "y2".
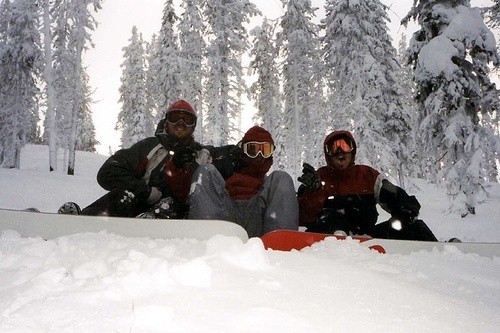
[
  {"x1": 298, "y1": 163, "x2": 320, "y2": 189},
  {"x1": 405, "y1": 196, "x2": 421, "y2": 216},
  {"x1": 149, "y1": 187, "x2": 163, "y2": 205}
]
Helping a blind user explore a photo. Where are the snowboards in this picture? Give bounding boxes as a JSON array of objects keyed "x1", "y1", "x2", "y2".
[
  {"x1": 0, "y1": 208, "x2": 249, "y2": 244},
  {"x1": 261, "y1": 230, "x2": 500, "y2": 260}
]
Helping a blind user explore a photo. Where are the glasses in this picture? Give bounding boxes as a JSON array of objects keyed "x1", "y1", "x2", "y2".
[
  {"x1": 166, "y1": 112, "x2": 196, "y2": 127},
  {"x1": 242, "y1": 141, "x2": 274, "y2": 158},
  {"x1": 325, "y1": 135, "x2": 354, "y2": 156}
]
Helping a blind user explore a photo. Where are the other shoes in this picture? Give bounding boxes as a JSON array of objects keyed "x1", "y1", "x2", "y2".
[
  {"x1": 59, "y1": 202, "x2": 81, "y2": 216},
  {"x1": 138, "y1": 213, "x2": 155, "y2": 222}
]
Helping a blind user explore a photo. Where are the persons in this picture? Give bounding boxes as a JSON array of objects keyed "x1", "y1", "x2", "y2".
[
  {"x1": 58, "y1": 100, "x2": 215, "y2": 220},
  {"x1": 296, "y1": 131, "x2": 462, "y2": 243},
  {"x1": 189, "y1": 126, "x2": 299, "y2": 239}
]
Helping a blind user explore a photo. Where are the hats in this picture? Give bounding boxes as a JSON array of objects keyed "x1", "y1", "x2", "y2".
[
  {"x1": 166, "y1": 100, "x2": 194, "y2": 113},
  {"x1": 242, "y1": 126, "x2": 274, "y2": 144}
]
